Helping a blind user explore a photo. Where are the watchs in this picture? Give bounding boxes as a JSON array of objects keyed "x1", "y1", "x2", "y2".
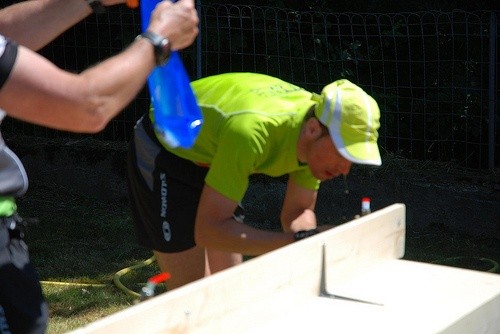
[{"x1": 140, "y1": 32, "x2": 169, "y2": 65}]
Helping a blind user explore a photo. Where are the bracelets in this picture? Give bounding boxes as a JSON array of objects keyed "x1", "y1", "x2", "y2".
[
  {"x1": 294, "y1": 230, "x2": 319, "y2": 240},
  {"x1": 85, "y1": 0, "x2": 103, "y2": 14}
]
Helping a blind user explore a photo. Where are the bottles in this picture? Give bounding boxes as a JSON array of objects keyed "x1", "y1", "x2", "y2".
[{"x1": 361, "y1": 198, "x2": 372, "y2": 216}]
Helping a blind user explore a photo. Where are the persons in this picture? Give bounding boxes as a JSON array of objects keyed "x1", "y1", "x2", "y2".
[
  {"x1": 121, "y1": 72, "x2": 383, "y2": 289},
  {"x1": 0, "y1": 0, "x2": 200, "y2": 334}
]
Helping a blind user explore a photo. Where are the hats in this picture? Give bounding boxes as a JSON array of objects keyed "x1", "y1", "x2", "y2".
[{"x1": 311, "y1": 79, "x2": 383, "y2": 166}]
